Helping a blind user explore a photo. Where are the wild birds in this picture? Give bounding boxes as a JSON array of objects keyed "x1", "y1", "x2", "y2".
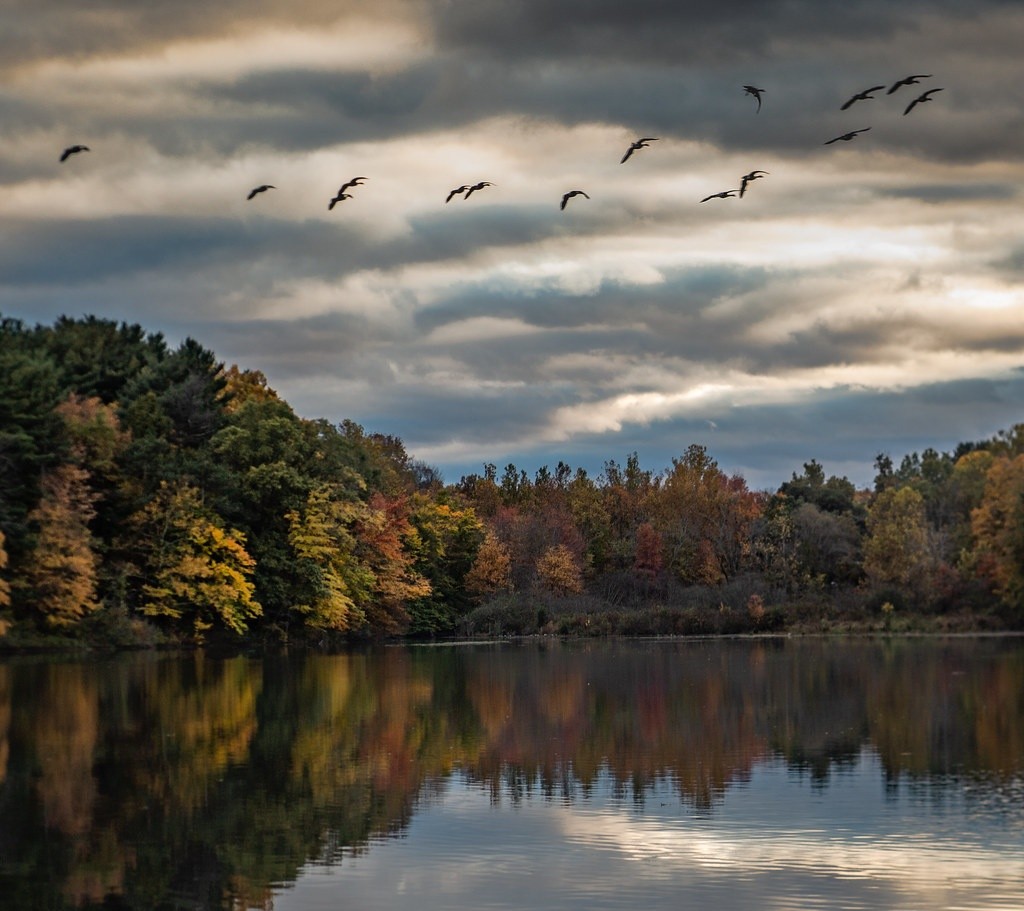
[
  {"x1": 445, "y1": 184, "x2": 471, "y2": 204},
  {"x1": 823, "y1": 127, "x2": 872, "y2": 145},
  {"x1": 743, "y1": 85, "x2": 766, "y2": 113},
  {"x1": 561, "y1": 190, "x2": 590, "y2": 210},
  {"x1": 840, "y1": 84, "x2": 886, "y2": 111},
  {"x1": 247, "y1": 185, "x2": 277, "y2": 201},
  {"x1": 465, "y1": 181, "x2": 497, "y2": 200},
  {"x1": 339, "y1": 176, "x2": 369, "y2": 193},
  {"x1": 700, "y1": 190, "x2": 741, "y2": 203},
  {"x1": 740, "y1": 170, "x2": 770, "y2": 199},
  {"x1": 619, "y1": 138, "x2": 659, "y2": 164},
  {"x1": 59, "y1": 144, "x2": 91, "y2": 163},
  {"x1": 903, "y1": 87, "x2": 945, "y2": 116},
  {"x1": 886, "y1": 74, "x2": 933, "y2": 95},
  {"x1": 327, "y1": 193, "x2": 353, "y2": 211}
]
